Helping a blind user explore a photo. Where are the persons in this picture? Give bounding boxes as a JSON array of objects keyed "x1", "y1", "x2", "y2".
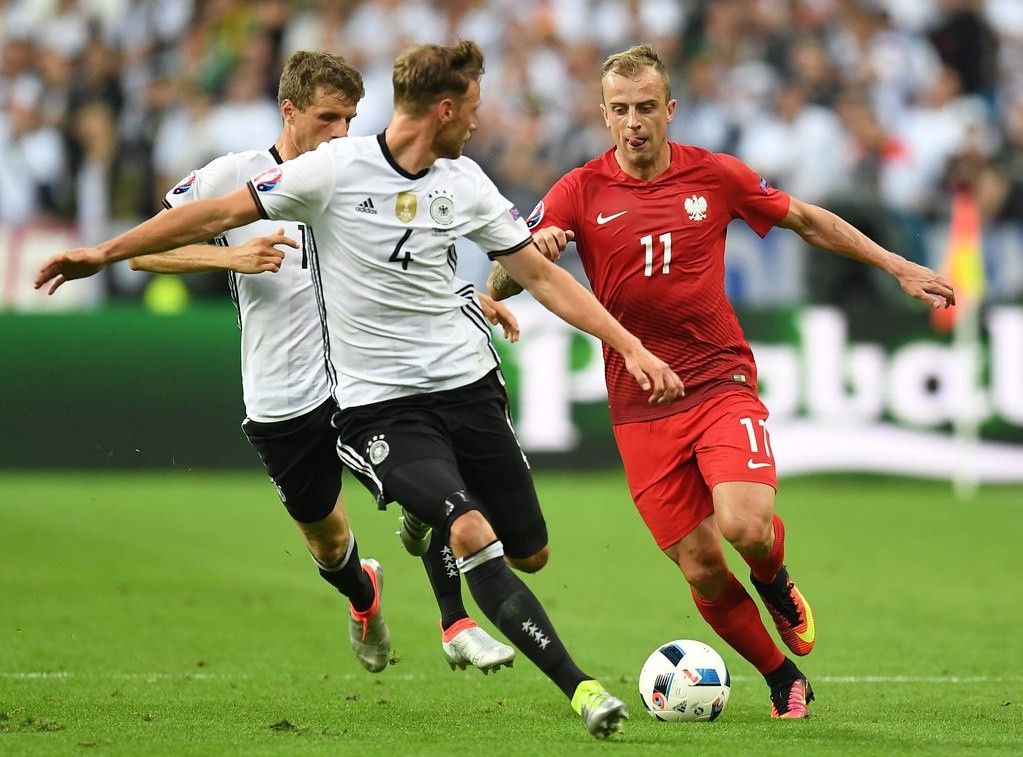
[
  {"x1": 34, "y1": 38, "x2": 686, "y2": 743},
  {"x1": 127, "y1": 49, "x2": 515, "y2": 675},
  {"x1": 2, "y1": 1, "x2": 1023, "y2": 310},
  {"x1": 487, "y1": 43, "x2": 957, "y2": 719}
]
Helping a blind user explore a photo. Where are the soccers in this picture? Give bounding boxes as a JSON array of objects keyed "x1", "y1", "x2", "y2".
[{"x1": 638, "y1": 638, "x2": 732, "y2": 724}]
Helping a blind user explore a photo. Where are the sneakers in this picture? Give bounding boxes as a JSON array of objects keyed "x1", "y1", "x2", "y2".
[
  {"x1": 569, "y1": 679, "x2": 628, "y2": 737},
  {"x1": 770, "y1": 672, "x2": 814, "y2": 719},
  {"x1": 440, "y1": 616, "x2": 516, "y2": 675},
  {"x1": 394, "y1": 507, "x2": 432, "y2": 556},
  {"x1": 750, "y1": 568, "x2": 815, "y2": 656},
  {"x1": 348, "y1": 559, "x2": 390, "y2": 672}
]
